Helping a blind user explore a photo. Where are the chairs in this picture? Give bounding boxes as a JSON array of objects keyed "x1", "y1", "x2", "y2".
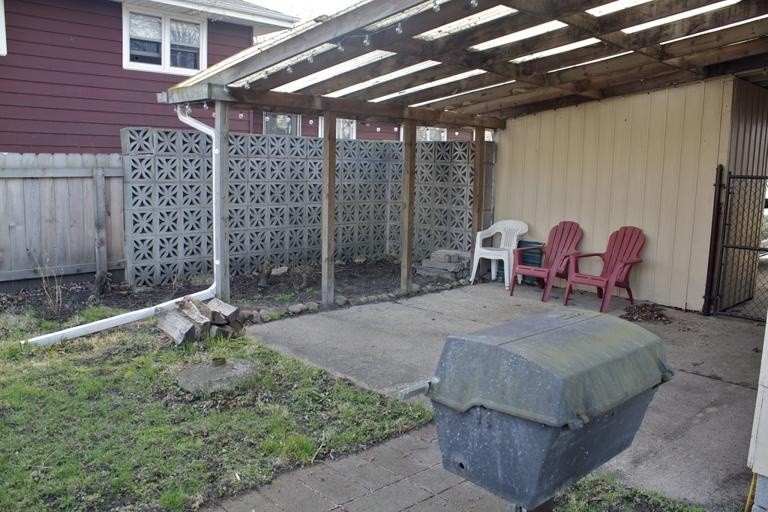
[{"x1": 469, "y1": 219, "x2": 646, "y2": 315}]
